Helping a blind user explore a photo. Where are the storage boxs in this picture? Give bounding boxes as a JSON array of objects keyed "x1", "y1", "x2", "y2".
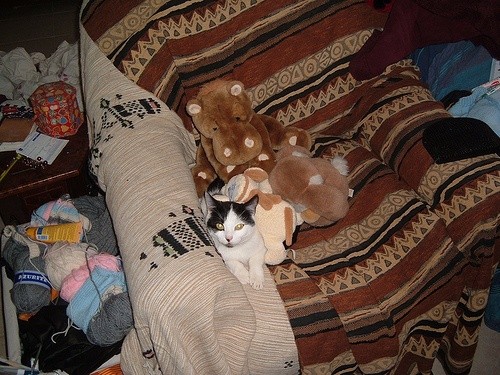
[
  {"x1": 28, "y1": 81, "x2": 84, "y2": 139},
  {"x1": 1, "y1": 220, "x2": 132, "y2": 375}
]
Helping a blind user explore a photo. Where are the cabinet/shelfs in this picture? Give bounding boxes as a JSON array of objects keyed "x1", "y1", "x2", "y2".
[{"x1": 0, "y1": 104, "x2": 95, "y2": 226}]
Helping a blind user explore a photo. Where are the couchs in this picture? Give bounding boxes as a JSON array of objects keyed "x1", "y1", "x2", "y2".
[{"x1": 78, "y1": 0, "x2": 500, "y2": 374}]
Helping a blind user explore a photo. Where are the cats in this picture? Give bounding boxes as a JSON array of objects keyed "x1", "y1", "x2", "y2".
[{"x1": 199, "y1": 176, "x2": 267, "y2": 290}]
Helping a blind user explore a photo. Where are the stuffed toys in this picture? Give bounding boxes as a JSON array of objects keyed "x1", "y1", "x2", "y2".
[
  {"x1": 184, "y1": 77, "x2": 312, "y2": 199},
  {"x1": 219, "y1": 146, "x2": 354, "y2": 265}
]
